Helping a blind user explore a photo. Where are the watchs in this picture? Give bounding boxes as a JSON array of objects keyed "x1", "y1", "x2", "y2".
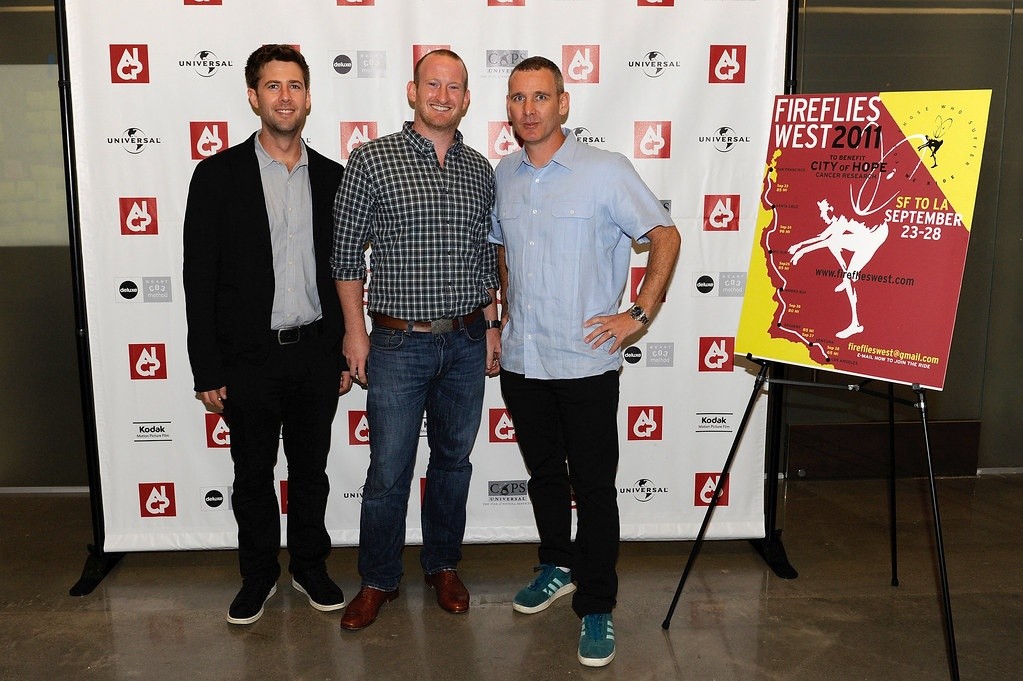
[{"x1": 629, "y1": 304, "x2": 652, "y2": 325}]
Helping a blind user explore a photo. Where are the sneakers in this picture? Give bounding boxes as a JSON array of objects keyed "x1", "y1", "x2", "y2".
[
  {"x1": 227, "y1": 577, "x2": 277, "y2": 624},
  {"x1": 512, "y1": 564, "x2": 577, "y2": 614},
  {"x1": 577, "y1": 613, "x2": 616, "y2": 667},
  {"x1": 292, "y1": 561, "x2": 346, "y2": 612}
]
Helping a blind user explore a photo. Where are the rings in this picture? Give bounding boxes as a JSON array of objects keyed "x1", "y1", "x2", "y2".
[
  {"x1": 608, "y1": 331, "x2": 613, "y2": 336},
  {"x1": 218, "y1": 398, "x2": 221, "y2": 400},
  {"x1": 494, "y1": 358, "x2": 498, "y2": 362}
]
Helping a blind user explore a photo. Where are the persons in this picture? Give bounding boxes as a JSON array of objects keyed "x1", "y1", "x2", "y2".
[
  {"x1": 182, "y1": 44, "x2": 353, "y2": 625},
  {"x1": 487, "y1": 55, "x2": 682, "y2": 667},
  {"x1": 329, "y1": 49, "x2": 502, "y2": 630}
]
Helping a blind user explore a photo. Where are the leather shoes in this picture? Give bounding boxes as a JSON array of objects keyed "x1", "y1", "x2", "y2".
[
  {"x1": 340, "y1": 585, "x2": 399, "y2": 630},
  {"x1": 424, "y1": 569, "x2": 469, "y2": 614}
]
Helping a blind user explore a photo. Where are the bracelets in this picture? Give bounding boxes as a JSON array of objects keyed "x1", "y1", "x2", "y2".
[{"x1": 485, "y1": 319, "x2": 501, "y2": 329}]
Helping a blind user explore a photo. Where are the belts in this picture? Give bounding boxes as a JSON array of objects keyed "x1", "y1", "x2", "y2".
[
  {"x1": 372, "y1": 308, "x2": 482, "y2": 334},
  {"x1": 260, "y1": 317, "x2": 325, "y2": 347}
]
